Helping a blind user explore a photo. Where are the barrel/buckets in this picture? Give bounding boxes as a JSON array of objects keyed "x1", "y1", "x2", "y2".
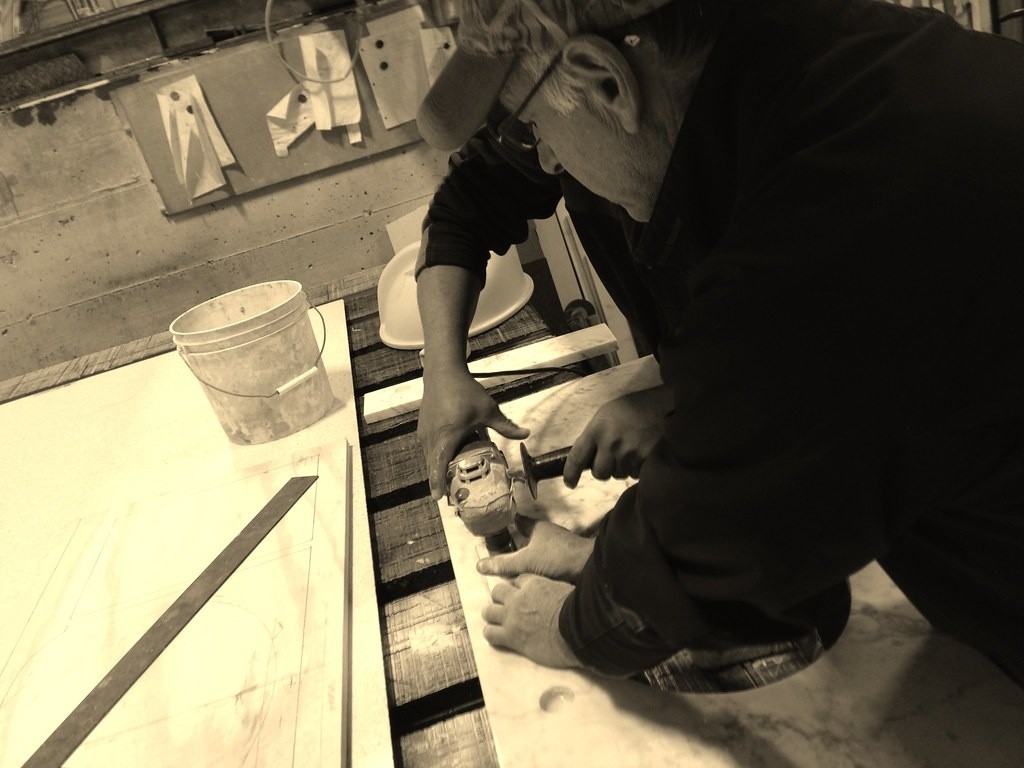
[{"x1": 168, "y1": 279, "x2": 334, "y2": 447}]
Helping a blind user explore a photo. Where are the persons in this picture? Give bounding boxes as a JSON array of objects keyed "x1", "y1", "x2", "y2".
[{"x1": 415, "y1": 1, "x2": 1024, "y2": 693}]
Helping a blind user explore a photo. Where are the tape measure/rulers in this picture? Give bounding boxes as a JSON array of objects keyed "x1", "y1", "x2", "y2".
[{"x1": 20, "y1": 471, "x2": 325, "y2": 768}]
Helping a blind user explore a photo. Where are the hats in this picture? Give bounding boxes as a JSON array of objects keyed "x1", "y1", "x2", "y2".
[{"x1": 416, "y1": 0, "x2": 664, "y2": 150}]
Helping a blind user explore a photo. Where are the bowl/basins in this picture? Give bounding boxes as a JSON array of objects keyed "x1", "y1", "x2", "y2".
[{"x1": 376, "y1": 238, "x2": 535, "y2": 349}]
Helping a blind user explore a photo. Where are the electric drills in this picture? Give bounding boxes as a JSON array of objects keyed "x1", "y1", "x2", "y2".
[{"x1": 442, "y1": 424, "x2": 596, "y2": 560}]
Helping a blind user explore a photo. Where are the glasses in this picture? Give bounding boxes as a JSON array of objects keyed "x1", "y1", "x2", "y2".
[{"x1": 496, "y1": 35, "x2": 640, "y2": 147}]
